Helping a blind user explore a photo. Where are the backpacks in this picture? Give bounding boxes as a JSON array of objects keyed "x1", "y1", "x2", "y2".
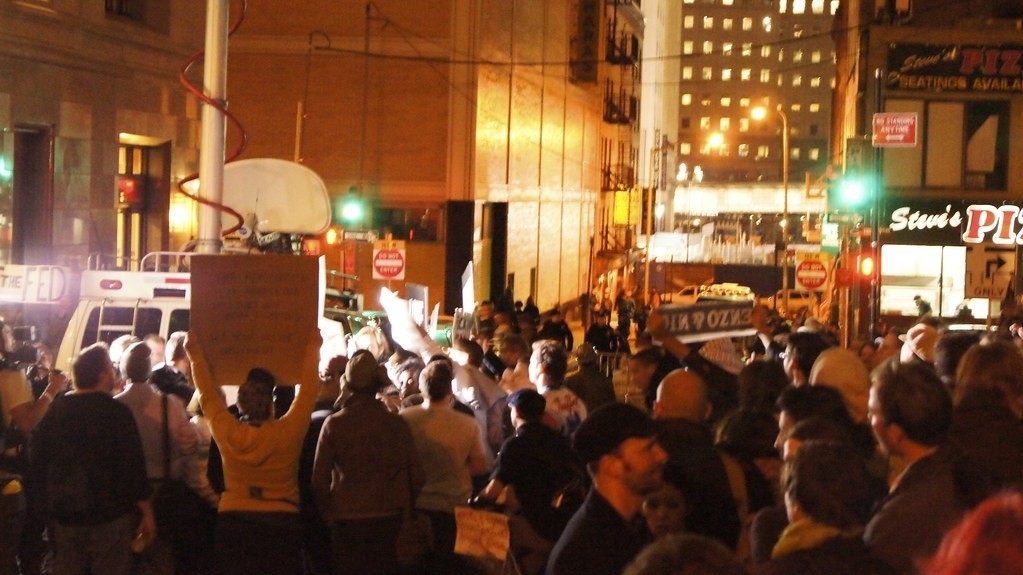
[{"x1": 521, "y1": 431, "x2": 580, "y2": 529}]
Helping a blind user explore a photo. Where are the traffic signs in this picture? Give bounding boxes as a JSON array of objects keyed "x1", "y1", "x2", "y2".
[{"x1": 962, "y1": 243, "x2": 1017, "y2": 299}]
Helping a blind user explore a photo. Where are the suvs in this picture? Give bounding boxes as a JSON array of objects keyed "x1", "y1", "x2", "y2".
[{"x1": 756, "y1": 288, "x2": 828, "y2": 317}]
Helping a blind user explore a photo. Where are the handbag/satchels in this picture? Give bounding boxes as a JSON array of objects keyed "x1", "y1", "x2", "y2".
[{"x1": 159, "y1": 477, "x2": 185, "y2": 520}]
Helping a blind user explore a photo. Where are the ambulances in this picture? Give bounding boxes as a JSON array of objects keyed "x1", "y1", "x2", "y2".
[{"x1": 47, "y1": 251, "x2": 392, "y2": 394}]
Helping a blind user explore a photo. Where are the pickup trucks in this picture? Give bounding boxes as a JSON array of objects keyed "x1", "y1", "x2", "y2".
[{"x1": 660, "y1": 285, "x2": 756, "y2": 307}]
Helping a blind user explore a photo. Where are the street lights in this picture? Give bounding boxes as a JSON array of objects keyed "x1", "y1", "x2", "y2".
[{"x1": 751, "y1": 102, "x2": 791, "y2": 317}]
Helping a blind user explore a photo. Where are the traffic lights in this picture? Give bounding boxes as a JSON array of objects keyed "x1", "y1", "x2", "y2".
[
  {"x1": 838, "y1": 173, "x2": 869, "y2": 209},
  {"x1": 340, "y1": 198, "x2": 362, "y2": 225},
  {"x1": 860, "y1": 255, "x2": 876, "y2": 279}
]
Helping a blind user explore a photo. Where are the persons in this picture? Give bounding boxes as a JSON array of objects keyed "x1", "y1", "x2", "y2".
[{"x1": 0, "y1": 285, "x2": 1023, "y2": 575}]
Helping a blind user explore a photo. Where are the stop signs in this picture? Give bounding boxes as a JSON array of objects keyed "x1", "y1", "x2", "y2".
[
  {"x1": 371, "y1": 238, "x2": 405, "y2": 281},
  {"x1": 794, "y1": 249, "x2": 830, "y2": 294}
]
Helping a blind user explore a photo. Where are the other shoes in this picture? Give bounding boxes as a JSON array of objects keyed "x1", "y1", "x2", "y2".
[{"x1": 21, "y1": 553, "x2": 52, "y2": 573}]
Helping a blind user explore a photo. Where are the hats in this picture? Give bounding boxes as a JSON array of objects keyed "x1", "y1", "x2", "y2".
[
  {"x1": 342, "y1": 350, "x2": 391, "y2": 391},
  {"x1": 898, "y1": 323, "x2": 940, "y2": 364},
  {"x1": 574, "y1": 343, "x2": 597, "y2": 363},
  {"x1": 505, "y1": 390, "x2": 548, "y2": 415},
  {"x1": 571, "y1": 402, "x2": 660, "y2": 459}
]
